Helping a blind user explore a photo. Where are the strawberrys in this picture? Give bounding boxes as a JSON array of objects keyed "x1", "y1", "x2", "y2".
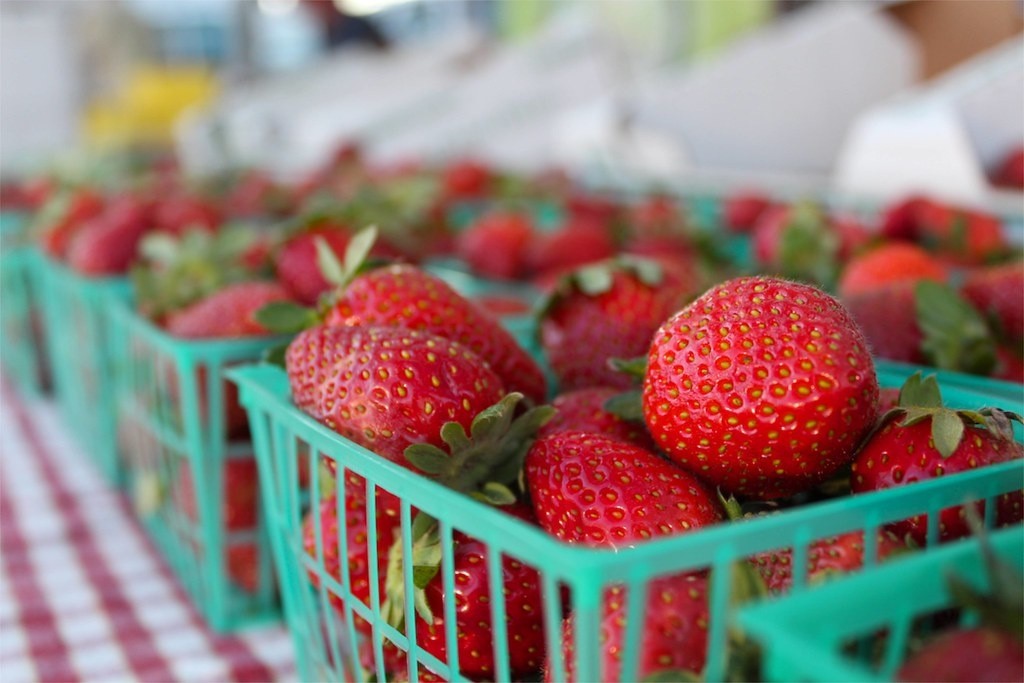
[{"x1": 0, "y1": 143, "x2": 1024, "y2": 683}]
